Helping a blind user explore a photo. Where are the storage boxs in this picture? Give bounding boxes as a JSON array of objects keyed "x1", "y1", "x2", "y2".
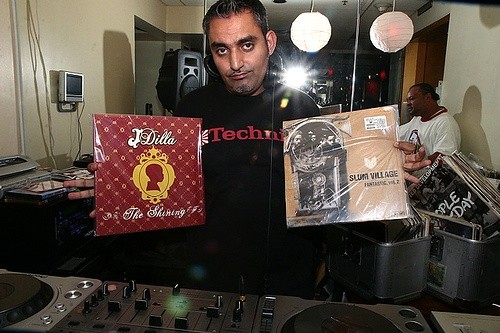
[{"x1": 326, "y1": 210, "x2": 500, "y2": 311}]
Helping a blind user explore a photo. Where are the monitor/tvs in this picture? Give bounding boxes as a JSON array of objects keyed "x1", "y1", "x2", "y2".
[{"x1": 58, "y1": 71, "x2": 85, "y2": 102}]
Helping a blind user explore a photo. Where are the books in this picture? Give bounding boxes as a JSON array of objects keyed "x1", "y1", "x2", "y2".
[
  {"x1": 404, "y1": 149, "x2": 500, "y2": 239},
  {"x1": 283, "y1": 105, "x2": 409, "y2": 228},
  {"x1": 92, "y1": 113, "x2": 205, "y2": 235}
]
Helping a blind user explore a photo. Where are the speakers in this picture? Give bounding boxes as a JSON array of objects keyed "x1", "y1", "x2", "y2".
[{"x1": 157, "y1": 50, "x2": 202, "y2": 109}]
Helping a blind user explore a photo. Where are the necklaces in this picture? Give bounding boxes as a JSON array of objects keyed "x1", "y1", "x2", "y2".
[{"x1": 430, "y1": 108, "x2": 444, "y2": 118}]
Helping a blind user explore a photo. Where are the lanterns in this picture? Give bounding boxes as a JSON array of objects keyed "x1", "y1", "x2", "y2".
[
  {"x1": 370, "y1": 11, "x2": 414, "y2": 53},
  {"x1": 290, "y1": 12, "x2": 331, "y2": 52}
]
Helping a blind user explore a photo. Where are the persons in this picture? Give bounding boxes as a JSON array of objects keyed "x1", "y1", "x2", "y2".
[
  {"x1": 400, "y1": 84, "x2": 461, "y2": 178},
  {"x1": 290, "y1": 127, "x2": 347, "y2": 208},
  {"x1": 63, "y1": 0, "x2": 431, "y2": 296},
  {"x1": 453, "y1": 180, "x2": 469, "y2": 197},
  {"x1": 423, "y1": 185, "x2": 439, "y2": 205}
]
